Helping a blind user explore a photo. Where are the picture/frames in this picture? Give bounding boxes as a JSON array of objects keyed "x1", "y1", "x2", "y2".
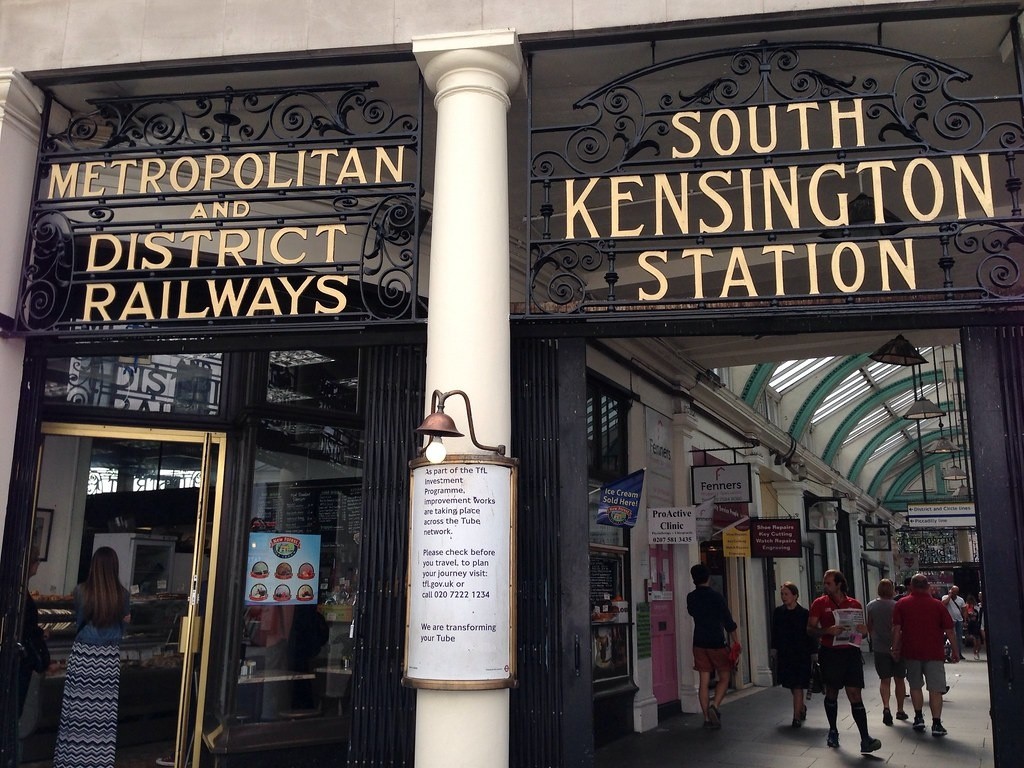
[{"x1": 35, "y1": 507, "x2": 54, "y2": 561}]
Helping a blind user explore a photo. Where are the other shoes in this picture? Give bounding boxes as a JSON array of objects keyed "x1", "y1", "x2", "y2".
[
  {"x1": 709, "y1": 705, "x2": 722, "y2": 728},
  {"x1": 861, "y1": 738, "x2": 882, "y2": 753},
  {"x1": 896, "y1": 711, "x2": 908, "y2": 720},
  {"x1": 883, "y1": 708, "x2": 893, "y2": 726},
  {"x1": 800, "y1": 705, "x2": 807, "y2": 720},
  {"x1": 704, "y1": 721, "x2": 713, "y2": 729},
  {"x1": 959, "y1": 653, "x2": 966, "y2": 660},
  {"x1": 974, "y1": 655, "x2": 979, "y2": 659},
  {"x1": 155, "y1": 753, "x2": 191, "y2": 766},
  {"x1": 827, "y1": 728, "x2": 840, "y2": 748},
  {"x1": 792, "y1": 719, "x2": 801, "y2": 728}
]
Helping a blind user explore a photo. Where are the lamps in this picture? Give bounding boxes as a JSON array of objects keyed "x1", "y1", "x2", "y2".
[
  {"x1": 415, "y1": 389, "x2": 507, "y2": 464},
  {"x1": 925, "y1": 346, "x2": 963, "y2": 454},
  {"x1": 820, "y1": 172, "x2": 906, "y2": 238},
  {"x1": 902, "y1": 347, "x2": 946, "y2": 420},
  {"x1": 867, "y1": 330, "x2": 929, "y2": 366},
  {"x1": 951, "y1": 382, "x2": 974, "y2": 496},
  {"x1": 941, "y1": 388, "x2": 967, "y2": 480}
]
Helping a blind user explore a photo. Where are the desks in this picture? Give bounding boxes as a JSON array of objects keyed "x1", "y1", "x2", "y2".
[
  {"x1": 315, "y1": 664, "x2": 352, "y2": 675},
  {"x1": 236, "y1": 669, "x2": 316, "y2": 723}
]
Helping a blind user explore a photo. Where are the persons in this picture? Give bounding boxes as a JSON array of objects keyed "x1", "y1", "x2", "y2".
[
  {"x1": 942, "y1": 585, "x2": 968, "y2": 659},
  {"x1": 18, "y1": 546, "x2": 50, "y2": 762},
  {"x1": 687, "y1": 564, "x2": 742, "y2": 729},
  {"x1": 809, "y1": 569, "x2": 882, "y2": 754},
  {"x1": 345, "y1": 567, "x2": 357, "y2": 584},
  {"x1": 966, "y1": 595, "x2": 986, "y2": 660},
  {"x1": 865, "y1": 577, "x2": 912, "y2": 726},
  {"x1": 254, "y1": 582, "x2": 338, "y2": 722},
  {"x1": 54, "y1": 546, "x2": 131, "y2": 768},
  {"x1": 889, "y1": 573, "x2": 960, "y2": 736},
  {"x1": 156, "y1": 569, "x2": 243, "y2": 767},
  {"x1": 768, "y1": 582, "x2": 819, "y2": 727}
]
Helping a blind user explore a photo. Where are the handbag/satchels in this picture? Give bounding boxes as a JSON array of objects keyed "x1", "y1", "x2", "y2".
[{"x1": 809, "y1": 661, "x2": 822, "y2": 694}]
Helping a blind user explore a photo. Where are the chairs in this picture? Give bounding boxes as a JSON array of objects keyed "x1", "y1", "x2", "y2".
[{"x1": 318, "y1": 658, "x2": 351, "y2": 716}]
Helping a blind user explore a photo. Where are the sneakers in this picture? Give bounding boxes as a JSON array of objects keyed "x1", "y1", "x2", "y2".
[
  {"x1": 932, "y1": 723, "x2": 948, "y2": 735},
  {"x1": 913, "y1": 718, "x2": 925, "y2": 728}
]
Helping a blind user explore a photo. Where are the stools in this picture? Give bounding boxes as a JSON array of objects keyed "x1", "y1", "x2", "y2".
[
  {"x1": 277, "y1": 708, "x2": 323, "y2": 722},
  {"x1": 237, "y1": 710, "x2": 256, "y2": 723}
]
